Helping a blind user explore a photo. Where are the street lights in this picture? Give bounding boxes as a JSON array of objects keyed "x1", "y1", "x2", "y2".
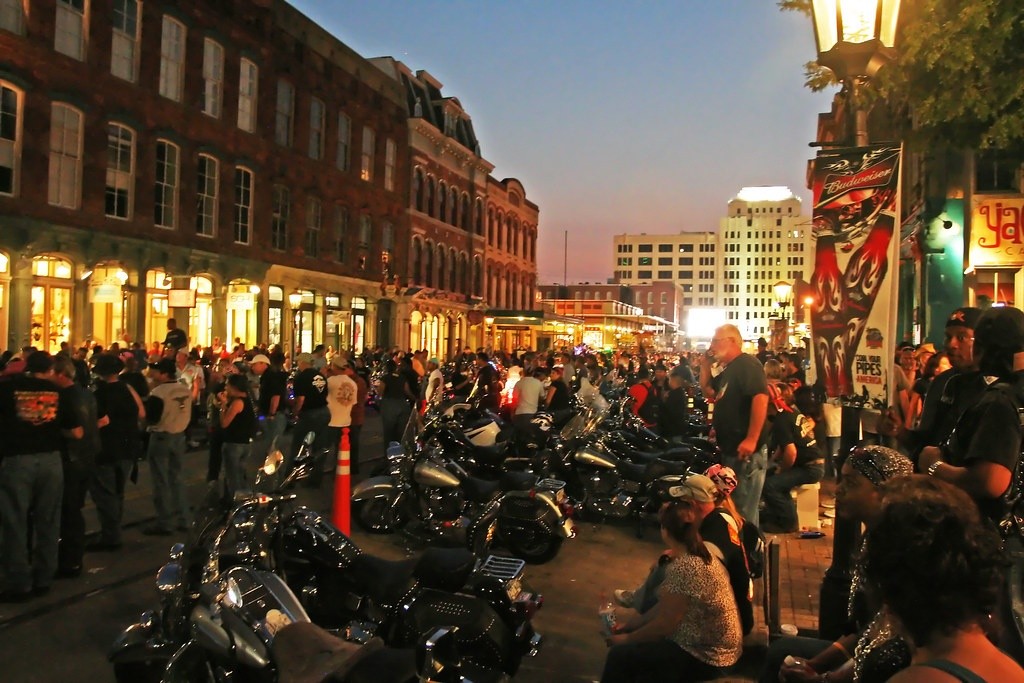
[{"x1": 807, "y1": 0, "x2": 902, "y2": 641}]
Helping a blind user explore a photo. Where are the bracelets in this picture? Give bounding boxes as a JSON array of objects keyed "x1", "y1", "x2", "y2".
[
  {"x1": 928, "y1": 461, "x2": 943, "y2": 477},
  {"x1": 219, "y1": 408, "x2": 225, "y2": 413},
  {"x1": 823, "y1": 672, "x2": 830, "y2": 683},
  {"x1": 268, "y1": 412, "x2": 277, "y2": 416}
]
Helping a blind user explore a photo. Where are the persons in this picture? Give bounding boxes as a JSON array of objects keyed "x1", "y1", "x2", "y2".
[
  {"x1": 163, "y1": 318, "x2": 187, "y2": 351},
  {"x1": 700, "y1": 324, "x2": 769, "y2": 531},
  {"x1": 0, "y1": 337, "x2": 699, "y2": 604},
  {"x1": 598, "y1": 464, "x2": 755, "y2": 683},
  {"x1": 756, "y1": 306, "x2": 1024, "y2": 683}
]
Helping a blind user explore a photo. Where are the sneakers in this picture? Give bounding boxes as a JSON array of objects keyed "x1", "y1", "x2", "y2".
[
  {"x1": 821, "y1": 498, "x2": 835, "y2": 507},
  {"x1": 824, "y1": 508, "x2": 835, "y2": 518}
]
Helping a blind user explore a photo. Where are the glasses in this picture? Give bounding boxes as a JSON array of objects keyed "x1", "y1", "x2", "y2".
[{"x1": 903, "y1": 348, "x2": 916, "y2": 352}]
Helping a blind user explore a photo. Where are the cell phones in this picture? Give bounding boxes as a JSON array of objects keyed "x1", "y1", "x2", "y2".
[{"x1": 706, "y1": 350, "x2": 713, "y2": 358}]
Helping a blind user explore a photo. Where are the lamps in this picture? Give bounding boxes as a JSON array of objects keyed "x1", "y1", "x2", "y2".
[
  {"x1": 161, "y1": 272, "x2": 173, "y2": 285},
  {"x1": 485, "y1": 315, "x2": 496, "y2": 324},
  {"x1": 933, "y1": 212, "x2": 952, "y2": 230},
  {"x1": 78, "y1": 264, "x2": 95, "y2": 281}
]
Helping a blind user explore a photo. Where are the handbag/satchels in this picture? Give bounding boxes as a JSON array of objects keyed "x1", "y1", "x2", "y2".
[{"x1": 1001, "y1": 514, "x2": 1024, "y2": 639}]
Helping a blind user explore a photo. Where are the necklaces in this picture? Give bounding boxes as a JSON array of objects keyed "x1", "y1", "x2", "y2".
[
  {"x1": 848, "y1": 539, "x2": 868, "y2": 617},
  {"x1": 854, "y1": 609, "x2": 894, "y2": 683}
]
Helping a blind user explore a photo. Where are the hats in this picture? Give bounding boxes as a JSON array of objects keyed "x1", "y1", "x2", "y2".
[
  {"x1": 331, "y1": 357, "x2": 347, "y2": 368},
  {"x1": 669, "y1": 475, "x2": 718, "y2": 502},
  {"x1": 845, "y1": 444, "x2": 913, "y2": 485},
  {"x1": 415, "y1": 350, "x2": 422, "y2": 354},
  {"x1": 178, "y1": 348, "x2": 189, "y2": 357},
  {"x1": 294, "y1": 353, "x2": 315, "y2": 364},
  {"x1": 945, "y1": 307, "x2": 985, "y2": 330},
  {"x1": 248, "y1": 354, "x2": 270, "y2": 365},
  {"x1": 148, "y1": 358, "x2": 176, "y2": 373},
  {"x1": 91, "y1": 355, "x2": 124, "y2": 375},
  {"x1": 429, "y1": 358, "x2": 438, "y2": 364},
  {"x1": 703, "y1": 464, "x2": 737, "y2": 497}
]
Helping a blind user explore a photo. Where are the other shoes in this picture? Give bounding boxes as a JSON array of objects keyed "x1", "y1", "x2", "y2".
[
  {"x1": 87, "y1": 540, "x2": 120, "y2": 552},
  {"x1": 615, "y1": 589, "x2": 630, "y2": 607},
  {"x1": 142, "y1": 527, "x2": 172, "y2": 535},
  {"x1": 176, "y1": 526, "x2": 187, "y2": 532}
]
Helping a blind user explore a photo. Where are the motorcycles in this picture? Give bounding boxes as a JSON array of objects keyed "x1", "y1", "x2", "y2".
[
  {"x1": 105, "y1": 427, "x2": 544, "y2": 683},
  {"x1": 350, "y1": 373, "x2": 722, "y2": 565}
]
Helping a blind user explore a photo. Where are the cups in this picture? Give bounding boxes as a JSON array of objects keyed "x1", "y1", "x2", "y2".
[{"x1": 599, "y1": 607, "x2": 616, "y2": 637}]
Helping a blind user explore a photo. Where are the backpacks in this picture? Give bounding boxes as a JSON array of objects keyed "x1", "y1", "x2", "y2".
[{"x1": 739, "y1": 516, "x2": 766, "y2": 580}]
